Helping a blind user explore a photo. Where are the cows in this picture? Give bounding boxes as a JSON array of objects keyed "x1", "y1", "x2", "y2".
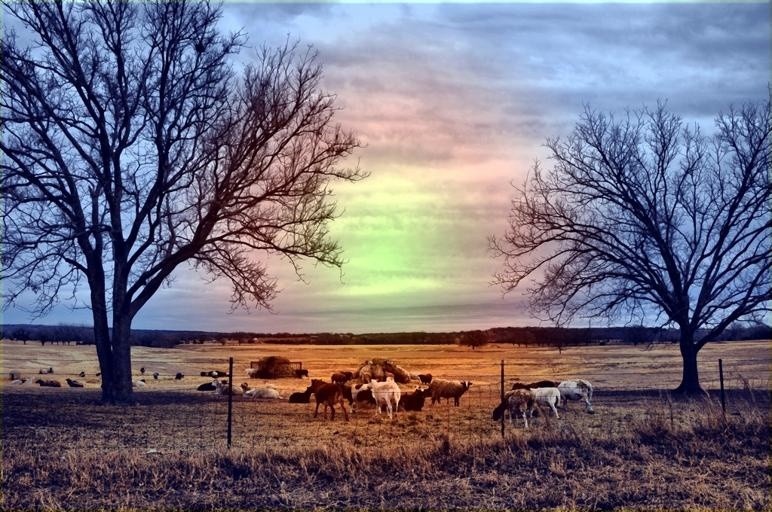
[
  {"x1": 197, "y1": 378, "x2": 284, "y2": 401},
  {"x1": 131, "y1": 379, "x2": 146, "y2": 390},
  {"x1": 287, "y1": 359, "x2": 433, "y2": 421},
  {"x1": 12, "y1": 378, "x2": 84, "y2": 387},
  {"x1": 491, "y1": 379, "x2": 596, "y2": 431},
  {"x1": 429, "y1": 380, "x2": 474, "y2": 407}
]
